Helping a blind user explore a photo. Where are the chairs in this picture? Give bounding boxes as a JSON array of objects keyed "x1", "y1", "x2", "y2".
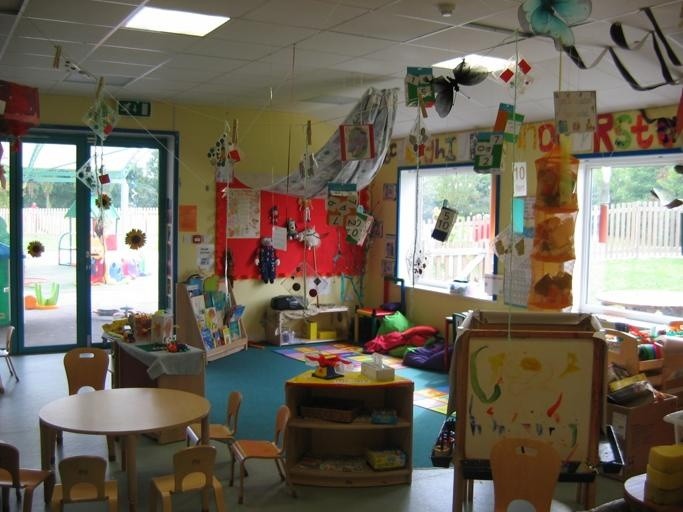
[
  {"x1": 485, "y1": 436, "x2": 563, "y2": 512},
  {"x1": 148, "y1": 446, "x2": 227, "y2": 512},
  {"x1": 0, "y1": 442, "x2": 55, "y2": 512},
  {"x1": 228, "y1": 404, "x2": 298, "y2": 506},
  {"x1": 184, "y1": 390, "x2": 249, "y2": 479},
  {"x1": 352, "y1": 276, "x2": 405, "y2": 346},
  {"x1": 0, "y1": 325, "x2": 20, "y2": 382},
  {"x1": 57, "y1": 346, "x2": 121, "y2": 463},
  {"x1": 48, "y1": 455, "x2": 120, "y2": 512}
]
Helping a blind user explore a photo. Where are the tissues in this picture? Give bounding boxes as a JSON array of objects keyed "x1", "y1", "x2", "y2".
[{"x1": 360, "y1": 352, "x2": 395, "y2": 382}]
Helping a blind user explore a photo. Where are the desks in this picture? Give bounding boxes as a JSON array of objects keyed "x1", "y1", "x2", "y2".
[{"x1": 37, "y1": 386, "x2": 212, "y2": 512}]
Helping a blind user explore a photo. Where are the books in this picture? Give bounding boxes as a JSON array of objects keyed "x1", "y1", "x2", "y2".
[
  {"x1": 320, "y1": 453, "x2": 367, "y2": 474},
  {"x1": 187, "y1": 275, "x2": 244, "y2": 349}
]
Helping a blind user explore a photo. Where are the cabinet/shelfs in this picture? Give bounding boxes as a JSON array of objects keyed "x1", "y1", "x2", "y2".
[
  {"x1": 283, "y1": 368, "x2": 414, "y2": 489},
  {"x1": 265, "y1": 306, "x2": 350, "y2": 347},
  {"x1": 110, "y1": 339, "x2": 207, "y2": 446}
]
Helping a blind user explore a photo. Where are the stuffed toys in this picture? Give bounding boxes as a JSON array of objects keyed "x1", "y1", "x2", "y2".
[
  {"x1": 268, "y1": 205, "x2": 279, "y2": 224},
  {"x1": 294, "y1": 225, "x2": 319, "y2": 249},
  {"x1": 285, "y1": 218, "x2": 298, "y2": 240},
  {"x1": 254, "y1": 236, "x2": 280, "y2": 285},
  {"x1": 298, "y1": 195, "x2": 312, "y2": 222}
]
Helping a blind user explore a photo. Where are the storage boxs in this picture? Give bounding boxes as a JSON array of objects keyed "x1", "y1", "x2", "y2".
[{"x1": 599, "y1": 391, "x2": 678, "y2": 483}]
